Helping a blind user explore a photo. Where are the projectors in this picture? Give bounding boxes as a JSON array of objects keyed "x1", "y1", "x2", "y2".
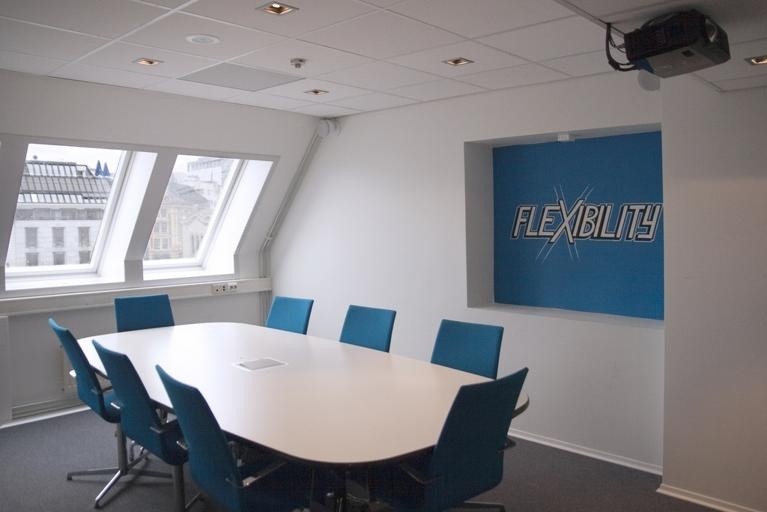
[{"x1": 624, "y1": 8, "x2": 731, "y2": 78}]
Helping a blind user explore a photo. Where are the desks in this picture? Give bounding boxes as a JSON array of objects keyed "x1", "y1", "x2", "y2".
[{"x1": 69, "y1": 319, "x2": 498, "y2": 510}]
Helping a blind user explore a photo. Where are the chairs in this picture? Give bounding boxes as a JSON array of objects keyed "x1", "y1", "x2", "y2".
[
  {"x1": 47, "y1": 317, "x2": 163, "y2": 507},
  {"x1": 93, "y1": 339, "x2": 192, "y2": 512},
  {"x1": 370, "y1": 367, "x2": 531, "y2": 511},
  {"x1": 157, "y1": 364, "x2": 312, "y2": 511},
  {"x1": 113, "y1": 295, "x2": 175, "y2": 332},
  {"x1": 265, "y1": 295, "x2": 314, "y2": 335},
  {"x1": 340, "y1": 304, "x2": 397, "y2": 353},
  {"x1": 430, "y1": 317, "x2": 505, "y2": 379}
]
